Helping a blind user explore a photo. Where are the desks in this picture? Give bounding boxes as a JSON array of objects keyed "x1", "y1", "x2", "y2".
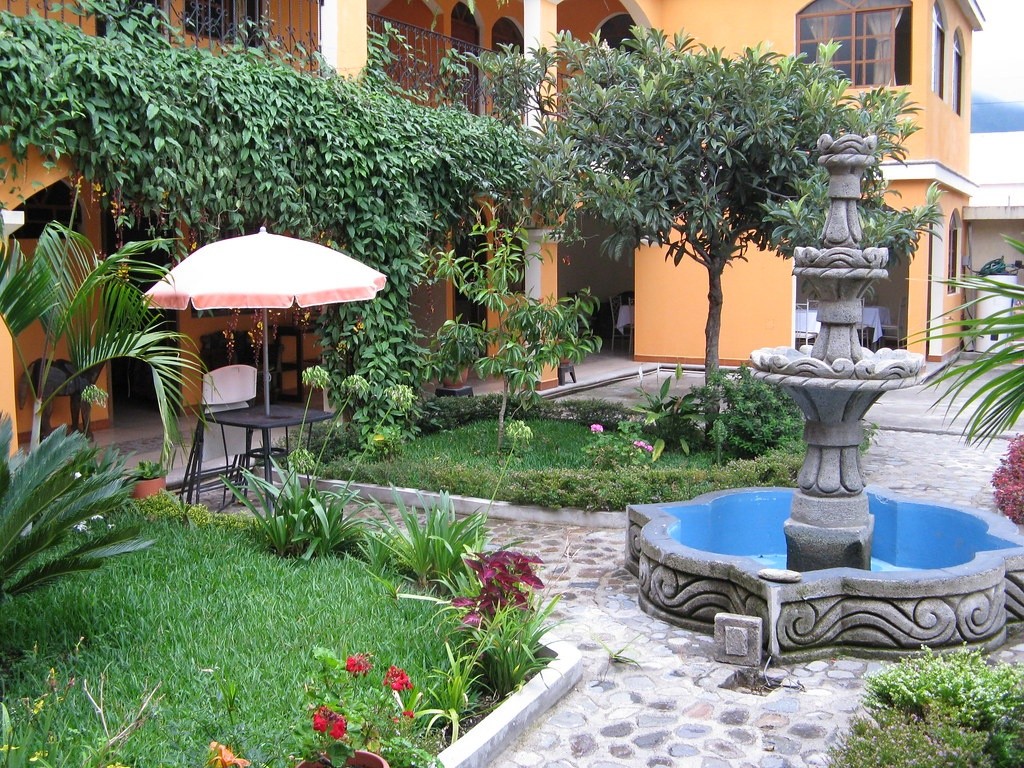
[
  {"x1": 181, "y1": 403, "x2": 333, "y2": 523},
  {"x1": 616, "y1": 305, "x2": 635, "y2": 335},
  {"x1": 796, "y1": 304, "x2": 891, "y2": 343}
]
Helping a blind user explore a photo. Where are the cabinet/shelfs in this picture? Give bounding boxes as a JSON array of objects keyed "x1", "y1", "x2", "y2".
[{"x1": 268, "y1": 323, "x2": 322, "y2": 403}]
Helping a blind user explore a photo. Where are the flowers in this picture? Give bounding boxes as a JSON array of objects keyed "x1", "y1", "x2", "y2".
[{"x1": 288, "y1": 646, "x2": 413, "y2": 768}]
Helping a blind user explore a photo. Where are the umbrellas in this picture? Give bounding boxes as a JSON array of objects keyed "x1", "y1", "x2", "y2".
[{"x1": 138, "y1": 227, "x2": 389, "y2": 417}]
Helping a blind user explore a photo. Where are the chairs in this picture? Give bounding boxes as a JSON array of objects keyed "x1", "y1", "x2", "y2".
[
  {"x1": 179, "y1": 364, "x2": 259, "y2": 512},
  {"x1": 199, "y1": 329, "x2": 284, "y2": 406},
  {"x1": 610, "y1": 294, "x2": 631, "y2": 351},
  {"x1": 795, "y1": 294, "x2": 907, "y2": 349},
  {"x1": 628, "y1": 297, "x2": 634, "y2": 354}
]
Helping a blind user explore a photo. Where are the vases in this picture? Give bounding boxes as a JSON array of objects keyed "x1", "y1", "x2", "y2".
[{"x1": 294, "y1": 750, "x2": 390, "y2": 768}]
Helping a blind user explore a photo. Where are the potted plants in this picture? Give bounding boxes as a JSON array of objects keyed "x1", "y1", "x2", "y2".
[
  {"x1": 433, "y1": 322, "x2": 469, "y2": 388},
  {"x1": 558, "y1": 328, "x2": 578, "y2": 363},
  {"x1": 129, "y1": 459, "x2": 169, "y2": 498}
]
